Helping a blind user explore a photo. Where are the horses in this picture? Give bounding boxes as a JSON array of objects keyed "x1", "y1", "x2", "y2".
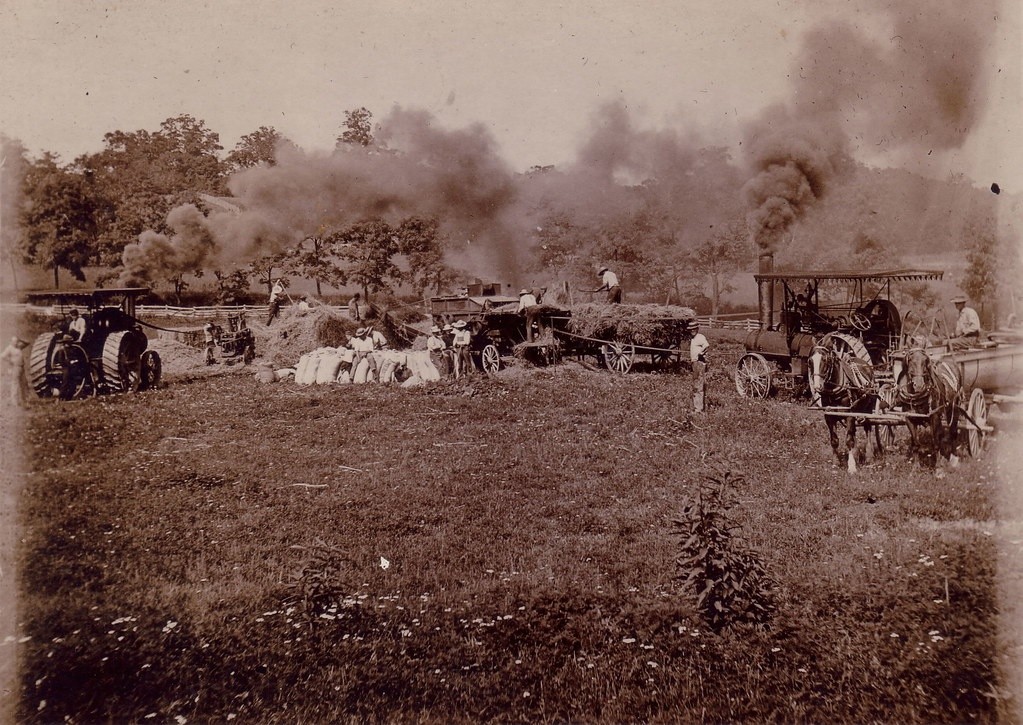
[
  {"x1": 891, "y1": 346, "x2": 963, "y2": 453},
  {"x1": 808, "y1": 330, "x2": 876, "y2": 469}
]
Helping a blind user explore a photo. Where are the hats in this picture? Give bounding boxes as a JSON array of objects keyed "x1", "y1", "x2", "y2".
[
  {"x1": 454, "y1": 320, "x2": 467, "y2": 327},
  {"x1": 598, "y1": 266, "x2": 608, "y2": 276},
  {"x1": 69, "y1": 309, "x2": 78, "y2": 316},
  {"x1": 272, "y1": 285, "x2": 283, "y2": 294},
  {"x1": 430, "y1": 326, "x2": 441, "y2": 333},
  {"x1": 365, "y1": 326, "x2": 374, "y2": 336},
  {"x1": 355, "y1": 327, "x2": 365, "y2": 336},
  {"x1": 204, "y1": 319, "x2": 213, "y2": 324},
  {"x1": 300, "y1": 295, "x2": 306, "y2": 298},
  {"x1": 443, "y1": 324, "x2": 453, "y2": 330},
  {"x1": 687, "y1": 321, "x2": 700, "y2": 329},
  {"x1": 519, "y1": 288, "x2": 530, "y2": 295},
  {"x1": 950, "y1": 294, "x2": 968, "y2": 302}
]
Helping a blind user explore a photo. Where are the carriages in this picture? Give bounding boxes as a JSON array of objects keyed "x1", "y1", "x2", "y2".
[
  {"x1": 535, "y1": 266, "x2": 702, "y2": 374},
  {"x1": 728, "y1": 267, "x2": 990, "y2": 481}
]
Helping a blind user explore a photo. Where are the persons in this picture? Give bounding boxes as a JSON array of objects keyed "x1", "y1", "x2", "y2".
[
  {"x1": 299, "y1": 295, "x2": 308, "y2": 307},
  {"x1": 2, "y1": 337, "x2": 31, "y2": 407},
  {"x1": 442, "y1": 326, "x2": 455, "y2": 349},
  {"x1": 686, "y1": 321, "x2": 710, "y2": 413},
  {"x1": 203, "y1": 318, "x2": 216, "y2": 347},
  {"x1": 949, "y1": 295, "x2": 980, "y2": 350},
  {"x1": 427, "y1": 326, "x2": 446, "y2": 378},
  {"x1": 366, "y1": 328, "x2": 387, "y2": 351},
  {"x1": 518, "y1": 288, "x2": 545, "y2": 343},
  {"x1": 266, "y1": 279, "x2": 282, "y2": 326},
  {"x1": 349, "y1": 327, "x2": 377, "y2": 381},
  {"x1": 452, "y1": 319, "x2": 473, "y2": 379},
  {"x1": 595, "y1": 267, "x2": 622, "y2": 304},
  {"x1": 349, "y1": 293, "x2": 362, "y2": 321},
  {"x1": 64, "y1": 309, "x2": 90, "y2": 343}
]
